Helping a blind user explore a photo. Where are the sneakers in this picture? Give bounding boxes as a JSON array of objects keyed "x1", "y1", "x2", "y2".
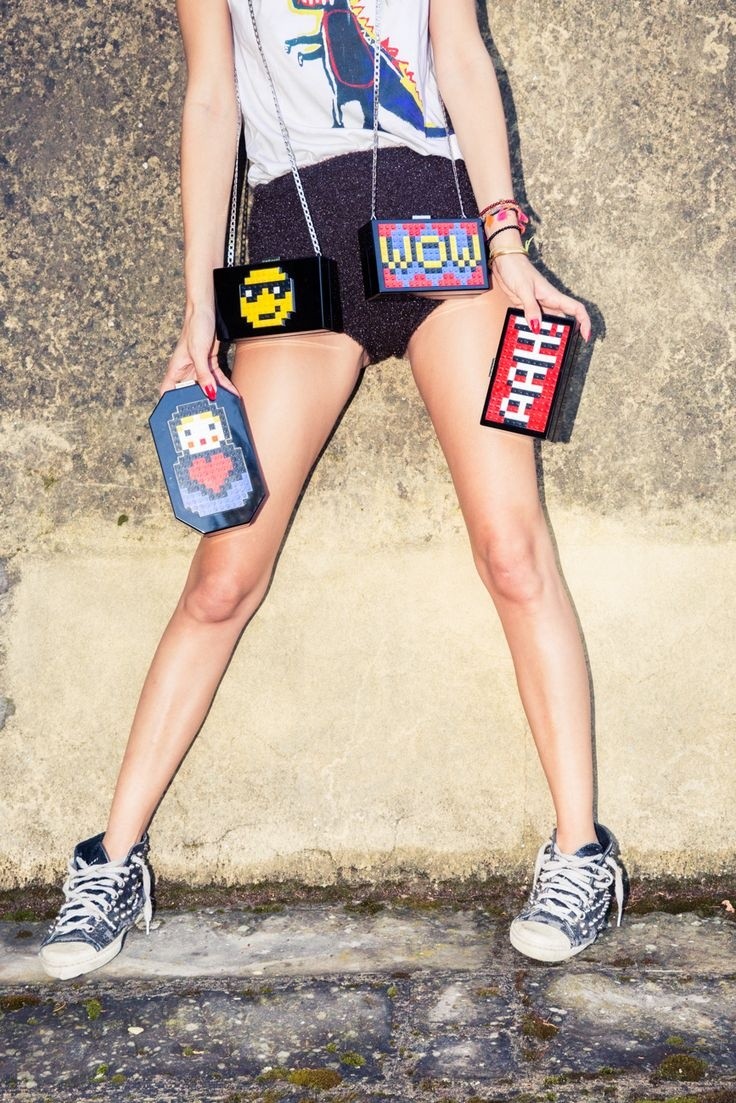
[
  {"x1": 38, "y1": 831, "x2": 153, "y2": 981},
  {"x1": 509, "y1": 820, "x2": 623, "y2": 964}
]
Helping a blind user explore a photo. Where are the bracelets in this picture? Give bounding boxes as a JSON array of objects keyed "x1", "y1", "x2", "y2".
[{"x1": 477, "y1": 199, "x2": 534, "y2": 269}]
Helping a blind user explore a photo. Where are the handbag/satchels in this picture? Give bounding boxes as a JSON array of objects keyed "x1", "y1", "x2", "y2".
[
  {"x1": 212, "y1": 259, "x2": 343, "y2": 343},
  {"x1": 359, "y1": 216, "x2": 489, "y2": 302}
]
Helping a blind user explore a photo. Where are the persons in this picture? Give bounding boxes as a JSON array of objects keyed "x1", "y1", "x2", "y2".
[{"x1": 39, "y1": 0, "x2": 623, "y2": 979}]
